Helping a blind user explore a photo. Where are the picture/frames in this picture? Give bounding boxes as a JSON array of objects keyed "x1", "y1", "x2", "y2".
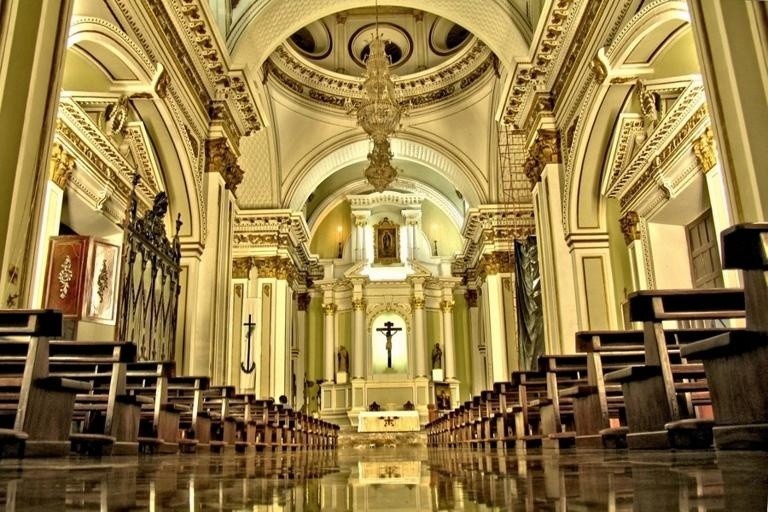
[
  {"x1": 80, "y1": 236, "x2": 125, "y2": 325},
  {"x1": 41, "y1": 235, "x2": 91, "y2": 319}
]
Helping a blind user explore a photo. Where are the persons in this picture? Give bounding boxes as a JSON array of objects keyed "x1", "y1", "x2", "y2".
[
  {"x1": 339, "y1": 346, "x2": 347, "y2": 372},
  {"x1": 379, "y1": 329, "x2": 398, "y2": 351},
  {"x1": 383, "y1": 232, "x2": 391, "y2": 250},
  {"x1": 279, "y1": 395, "x2": 291, "y2": 409},
  {"x1": 432, "y1": 343, "x2": 441, "y2": 369},
  {"x1": 436, "y1": 391, "x2": 451, "y2": 409}
]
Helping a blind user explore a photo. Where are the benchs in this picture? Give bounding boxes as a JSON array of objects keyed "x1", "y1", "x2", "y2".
[
  {"x1": 0, "y1": 308, "x2": 340, "y2": 454},
  {"x1": 0, "y1": 454, "x2": 340, "y2": 512},
  {"x1": 429, "y1": 448, "x2": 768, "y2": 511},
  {"x1": 425, "y1": 222, "x2": 767, "y2": 449}
]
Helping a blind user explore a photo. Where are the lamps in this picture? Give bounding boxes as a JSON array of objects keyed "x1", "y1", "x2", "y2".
[{"x1": 355, "y1": 0, "x2": 407, "y2": 193}]
